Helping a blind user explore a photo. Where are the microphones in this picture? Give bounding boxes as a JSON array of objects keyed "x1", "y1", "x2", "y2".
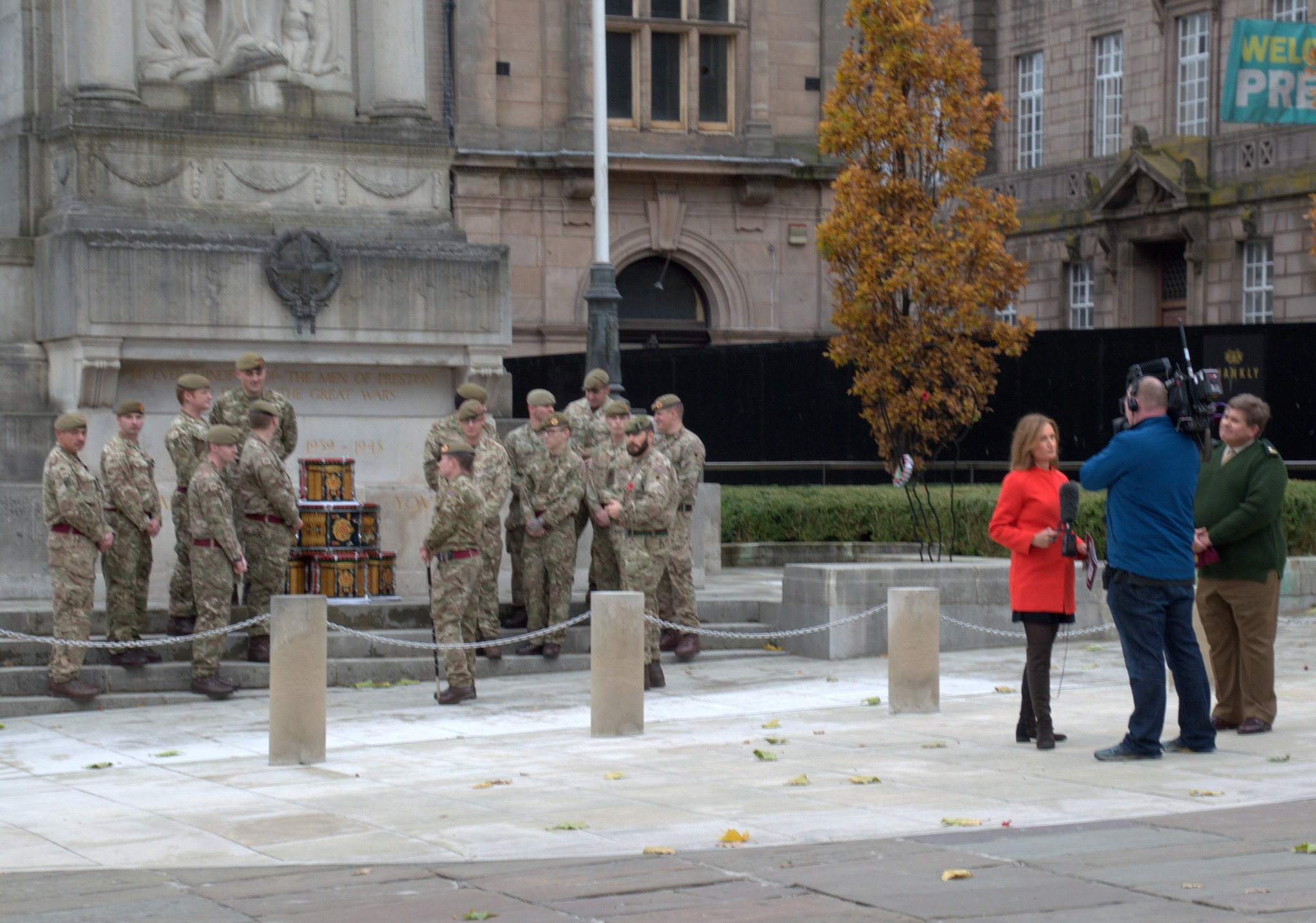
[{"x1": 1058, "y1": 481, "x2": 1080, "y2": 534}]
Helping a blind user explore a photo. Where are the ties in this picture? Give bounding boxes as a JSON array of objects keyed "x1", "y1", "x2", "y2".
[{"x1": 1221, "y1": 448, "x2": 1236, "y2": 465}]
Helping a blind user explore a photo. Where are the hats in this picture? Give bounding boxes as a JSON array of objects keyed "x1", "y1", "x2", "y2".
[
  {"x1": 55, "y1": 353, "x2": 281, "y2": 444},
  {"x1": 438, "y1": 370, "x2": 683, "y2": 456}
]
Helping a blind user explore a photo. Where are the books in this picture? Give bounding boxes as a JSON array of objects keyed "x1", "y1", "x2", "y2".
[{"x1": 1082, "y1": 530, "x2": 1098, "y2": 589}]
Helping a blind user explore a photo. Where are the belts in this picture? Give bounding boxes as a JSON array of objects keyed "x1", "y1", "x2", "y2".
[
  {"x1": 532, "y1": 509, "x2": 548, "y2": 517},
  {"x1": 193, "y1": 538, "x2": 219, "y2": 548},
  {"x1": 177, "y1": 486, "x2": 188, "y2": 493},
  {"x1": 52, "y1": 523, "x2": 85, "y2": 535},
  {"x1": 676, "y1": 504, "x2": 693, "y2": 513},
  {"x1": 106, "y1": 506, "x2": 152, "y2": 520},
  {"x1": 439, "y1": 548, "x2": 478, "y2": 563},
  {"x1": 245, "y1": 512, "x2": 287, "y2": 524},
  {"x1": 622, "y1": 527, "x2": 668, "y2": 536}
]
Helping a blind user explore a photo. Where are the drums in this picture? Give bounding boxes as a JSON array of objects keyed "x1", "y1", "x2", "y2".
[
  {"x1": 284, "y1": 559, "x2": 307, "y2": 594},
  {"x1": 289, "y1": 500, "x2": 382, "y2": 556},
  {"x1": 298, "y1": 456, "x2": 356, "y2": 502},
  {"x1": 308, "y1": 556, "x2": 368, "y2": 599},
  {"x1": 368, "y1": 552, "x2": 397, "y2": 598}
]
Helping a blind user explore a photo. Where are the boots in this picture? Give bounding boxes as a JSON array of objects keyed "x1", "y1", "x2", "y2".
[
  {"x1": 1016, "y1": 663, "x2": 1068, "y2": 744},
  {"x1": 1025, "y1": 645, "x2": 1055, "y2": 749}
]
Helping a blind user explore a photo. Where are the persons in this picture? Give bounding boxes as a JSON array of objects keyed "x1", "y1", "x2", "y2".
[
  {"x1": 165, "y1": 375, "x2": 213, "y2": 636},
  {"x1": 501, "y1": 389, "x2": 556, "y2": 628},
  {"x1": 239, "y1": 401, "x2": 303, "y2": 662},
  {"x1": 189, "y1": 425, "x2": 247, "y2": 694},
  {"x1": 562, "y1": 369, "x2": 615, "y2": 626},
  {"x1": 988, "y1": 414, "x2": 1089, "y2": 749},
  {"x1": 1079, "y1": 375, "x2": 1216, "y2": 760},
  {"x1": 646, "y1": 394, "x2": 705, "y2": 657},
  {"x1": 101, "y1": 402, "x2": 163, "y2": 667},
  {"x1": 604, "y1": 415, "x2": 680, "y2": 690},
  {"x1": 450, "y1": 400, "x2": 512, "y2": 658},
  {"x1": 418, "y1": 443, "x2": 486, "y2": 703},
  {"x1": 516, "y1": 412, "x2": 585, "y2": 658},
  {"x1": 41, "y1": 414, "x2": 117, "y2": 698},
  {"x1": 423, "y1": 384, "x2": 496, "y2": 655},
  {"x1": 584, "y1": 401, "x2": 633, "y2": 592},
  {"x1": 134, "y1": 0, "x2": 345, "y2": 80},
  {"x1": 209, "y1": 352, "x2": 299, "y2": 607},
  {"x1": 1192, "y1": 394, "x2": 1288, "y2": 733}
]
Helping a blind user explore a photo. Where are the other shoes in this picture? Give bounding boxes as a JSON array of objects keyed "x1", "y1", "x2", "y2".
[
  {"x1": 192, "y1": 671, "x2": 240, "y2": 696},
  {"x1": 659, "y1": 631, "x2": 676, "y2": 651},
  {"x1": 1236, "y1": 718, "x2": 1271, "y2": 734},
  {"x1": 1094, "y1": 745, "x2": 1162, "y2": 760},
  {"x1": 675, "y1": 634, "x2": 700, "y2": 655},
  {"x1": 167, "y1": 615, "x2": 195, "y2": 635},
  {"x1": 645, "y1": 664, "x2": 650, "y2": 691},
  {"x1": 433, "y1": 606, "x2": 561, "y2": 703},
  {"x1": 138, "y1": 647, "x2": 162, "y2": 664},
  {"x1": 111, "y1": 650, "x2": 141, "y2": 665},
  {"x1": 248, "y1": 637, "x2": 273, "y2": 661},
  {"x1": 649, "y1": 660, "x2": 665, "y2": 687},
  {"x1": 1210, "y1": 716, "x2": 1238, "y2": 729},
  {"x1": 49, "y1": 675, "x2": 100, "y2": 698},
  {"x1": 1164, "y1": 736, "x2": 1216, "y2": 752}
]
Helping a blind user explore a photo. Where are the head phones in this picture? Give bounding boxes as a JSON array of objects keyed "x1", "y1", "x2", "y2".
[{"x1": 1126, "y1": 373, "x2": 1148, "y2": 412}]
